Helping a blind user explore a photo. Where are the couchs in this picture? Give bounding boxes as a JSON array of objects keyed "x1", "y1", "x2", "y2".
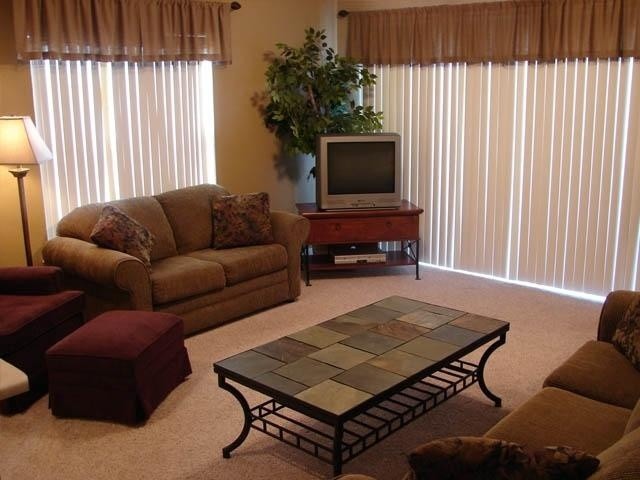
[
  {"x1": 486, "y1": 290, "x2": 640, "y2": 478},
  {"x1": 43, "y1": 184, "x2": 311, "y2": 337}
]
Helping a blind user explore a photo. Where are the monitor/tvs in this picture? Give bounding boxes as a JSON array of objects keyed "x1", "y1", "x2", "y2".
[{"x1": 315, "y1": 132, "x2": 403, "y2": 210}]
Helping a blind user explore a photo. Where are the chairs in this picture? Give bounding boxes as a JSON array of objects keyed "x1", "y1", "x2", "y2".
[{"x1": 0, "y1": 262, "x2": 85, "y2": 402}]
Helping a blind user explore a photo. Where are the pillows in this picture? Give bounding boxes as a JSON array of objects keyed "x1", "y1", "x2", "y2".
[
  {"x1": 406, "y1": 435, "x2": 599, "y2": 479},
  {"x1": 58, "y1": 198, "x2": 178, "y2": 261},
  {"x1": 211, "y1": 193, "x2": 275, "y2": 249},
  {"x1": 156, "y1": 183, "x2": 228, "y2": 255},
  {"x1": 612, "y1": 294, "x2": 639, "y2": 369},
  {"x1": 90, "y1": 206, "x2": 156, "y2": 275}
]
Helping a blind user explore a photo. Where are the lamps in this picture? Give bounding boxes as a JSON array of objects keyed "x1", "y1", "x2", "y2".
[{"x1": 0, "y1": 115, "x2": 54, "y2": 265}]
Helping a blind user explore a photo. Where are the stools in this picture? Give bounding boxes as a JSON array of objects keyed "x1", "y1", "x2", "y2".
[{"x1": 44, "y1": 309, "x2": 192, "y2": 427}]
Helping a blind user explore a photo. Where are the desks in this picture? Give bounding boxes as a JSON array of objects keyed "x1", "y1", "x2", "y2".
[{"x1": 296, "y1": 201, "x2": 423, "y2": 287}]
